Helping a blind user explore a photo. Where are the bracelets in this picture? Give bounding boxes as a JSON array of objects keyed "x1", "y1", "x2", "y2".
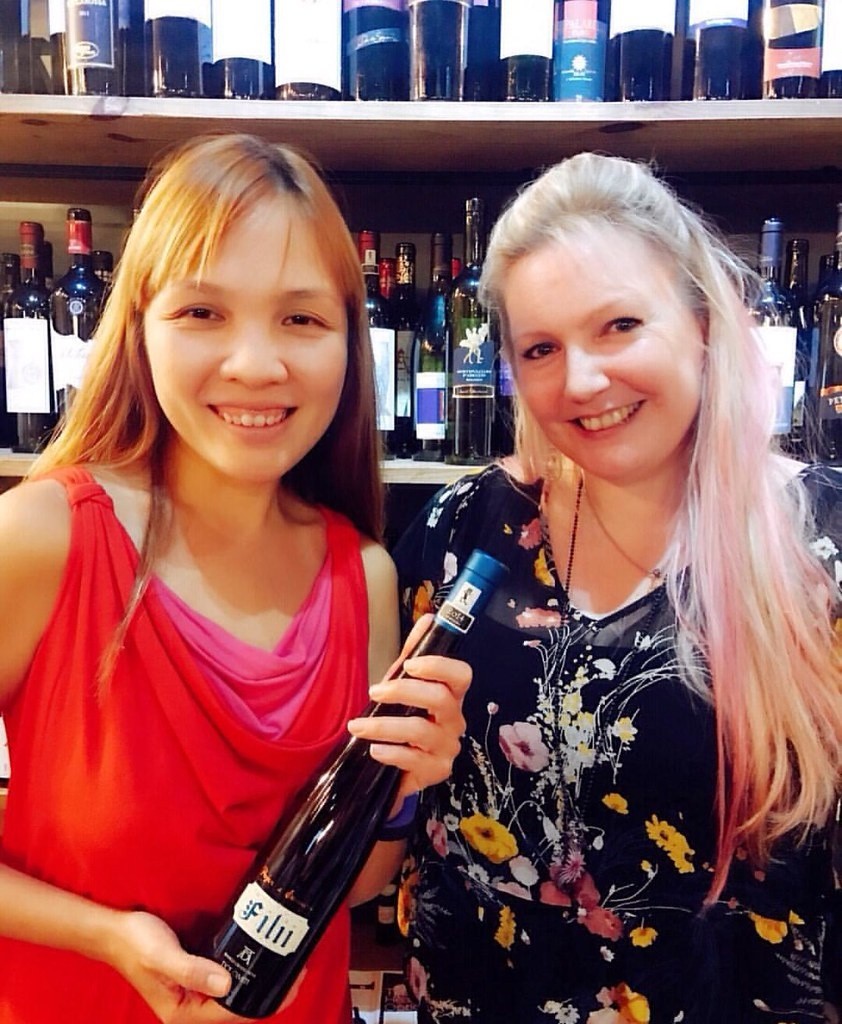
[{"x1": 374, "y1": 795, "x2": 418, "y2": 841}]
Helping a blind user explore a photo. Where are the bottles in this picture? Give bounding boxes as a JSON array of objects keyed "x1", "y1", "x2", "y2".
[
  {"x1": 750, "y1": 219, "x2": 807, "y2": 458},
  {"x1": 819, "y1": 203, "x2": 842, "y2": 466},
  {"x1": 809, "y1": 255, "x2": 833, "y2": 459},
  {"x1": 785, "y1": 239, "x2": 810, "y2": 453},
  {"x1": 0, "y1": 197, "x2": 523, "y2": 463},
  {"x1": 197, "y1": 549, "x2": 509, "y2": 1018}
]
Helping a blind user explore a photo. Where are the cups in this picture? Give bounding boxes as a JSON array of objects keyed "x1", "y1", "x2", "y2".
[{"x1": 0, "y1": 0, "x2": 842, "y2": 104}]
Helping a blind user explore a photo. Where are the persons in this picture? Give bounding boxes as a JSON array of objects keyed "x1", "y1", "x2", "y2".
[
  {"x1": 385, "y1": 154, "x2": 842, "y2": 1023},
  {"x1": 3, "y1": 137, "x2": 477, "y2": 1024}
]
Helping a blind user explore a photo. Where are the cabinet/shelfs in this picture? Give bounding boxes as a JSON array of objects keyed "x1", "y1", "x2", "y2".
[{"x1": 0, "y1": 91, "x2": 842, "y2": 487}]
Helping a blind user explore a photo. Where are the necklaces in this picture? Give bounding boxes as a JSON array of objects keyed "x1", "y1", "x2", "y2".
[{"x1": 553, "y1": 472, "x2": 676, "y2": 846}]
